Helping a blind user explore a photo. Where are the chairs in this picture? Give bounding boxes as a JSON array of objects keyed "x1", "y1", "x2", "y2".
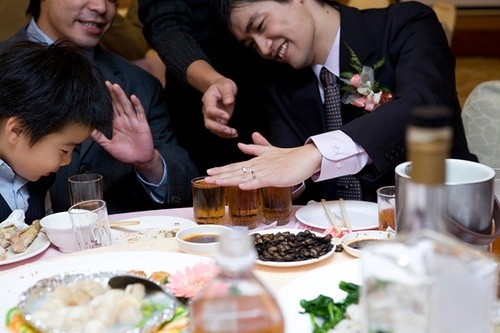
[{"x1": 461, "y1": 79, "x2": 500, "y2": 182}]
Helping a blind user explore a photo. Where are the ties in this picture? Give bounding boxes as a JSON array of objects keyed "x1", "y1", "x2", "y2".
[{"x1": 319, "y1": 66, "x2": 362, "y2": 201}]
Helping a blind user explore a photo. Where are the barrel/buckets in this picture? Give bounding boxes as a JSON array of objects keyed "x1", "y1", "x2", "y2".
[{"x1": 394, "y1": 159, "x2": 496, "y2": 255}]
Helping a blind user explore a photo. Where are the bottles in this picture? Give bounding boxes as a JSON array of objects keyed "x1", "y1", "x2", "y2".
[
  {"x1": 188, "y1": 227, "x2": 285, "y2": 333},
  {"x1": 357, "y1": 104, "x2": 497, "y2": 332}
]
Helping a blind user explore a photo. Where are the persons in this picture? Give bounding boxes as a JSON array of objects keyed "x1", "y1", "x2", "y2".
[
  {"x1": 204, "y1": 0, "x2": 479, "y2": 190},
  {"x1": 0, "y1": 36, "x2": 116, "y2": 225},
  {"x1": 138, "y1": 1, "x2": 273, "y2": 178},
  {"x1": 0, "y1": 0, "x2": 200, "y2": 215}
]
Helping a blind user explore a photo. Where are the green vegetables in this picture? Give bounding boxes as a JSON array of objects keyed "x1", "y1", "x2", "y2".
[{"x1": 298, "y1": 281, "x2": 363, "y2": 333}]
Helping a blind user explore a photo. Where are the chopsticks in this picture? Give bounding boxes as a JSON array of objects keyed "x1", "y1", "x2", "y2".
[
  {"x1": 109, "y1": 220, "x2": 141, "y2": 226},
  {"x1": 321, "y1": 198, "x2": 351, "y2": 234}
]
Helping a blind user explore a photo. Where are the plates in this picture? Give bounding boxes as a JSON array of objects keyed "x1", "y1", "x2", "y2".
[
  {"x1": 111, "y1": 214, "x2": 198, "y2": 248},
  {"x1": 296, "y1": 200, "x2": 379, "y2": 230},
  {"x1": 341, "y1": 230, "x2": 398, "y2": 258},
  {"x1": 176, "y1": 225, "x2": 235, "y2": 255},
  {"x1": 0, "y1": 251, "x2": 215, "y2": 333},
  {"x1": 247, "y1": 227, "x2": 334, "y2": 267},
  {"x1": 0, "y1": 232, "x2": 50, "y2": 266}
]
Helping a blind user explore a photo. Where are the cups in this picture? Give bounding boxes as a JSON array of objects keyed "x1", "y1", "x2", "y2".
[
  {"x1": 376, "y1": 187, "x2": 397, "y2": 232},
  {"x1": 226, "y1": 183, "x2": 262, "y2": 229},
  {"x1": 68, "y1": 173, "x2": 104, "y2": 207},
  {"x1": 190, "y1": 175, "x2": 226, "y2": 227},
  {"x1": 262, "y1": 186, "x2": 292, "y2": 227},
  {"x1": 67, "y1": 199, "x2": 113, "y2": 251}
]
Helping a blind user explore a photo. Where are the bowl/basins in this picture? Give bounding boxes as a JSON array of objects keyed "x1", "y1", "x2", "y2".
[{"x1": 39, "y1": 210, "x2": 98, "y2": 253}]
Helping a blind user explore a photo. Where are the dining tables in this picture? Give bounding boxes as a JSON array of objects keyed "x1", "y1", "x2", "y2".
[{"x1": 0, "y1": 205, "x2": 500, "y2": 333}]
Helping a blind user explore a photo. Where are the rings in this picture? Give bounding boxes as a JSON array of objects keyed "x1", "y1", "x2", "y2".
[
  {"x1": 242, "y1": 166, "x2": 247, "y2": 173},
  {"x1": 250, "y1": 171, "x2": 256, "y2": 179}
]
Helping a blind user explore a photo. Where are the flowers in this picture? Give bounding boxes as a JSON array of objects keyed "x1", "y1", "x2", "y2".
[{"x1": 341, "y1": 47, "x2": 392, "y2": 110}]
